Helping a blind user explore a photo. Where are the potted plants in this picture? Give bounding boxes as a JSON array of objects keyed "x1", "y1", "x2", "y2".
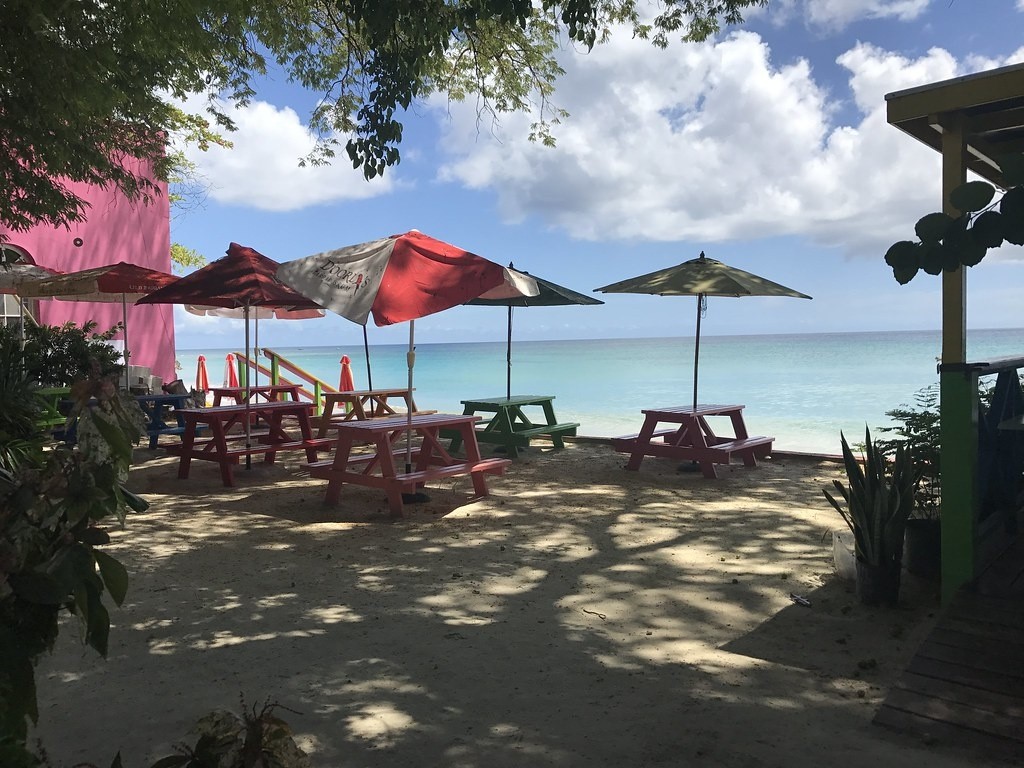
[{"x1": 821, "y1": 380, "x2": 942, "y2": 602}]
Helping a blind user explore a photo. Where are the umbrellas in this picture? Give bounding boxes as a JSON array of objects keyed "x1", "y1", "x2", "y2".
[
  {"x1": 267, "y1": 229, "x2": 605, "y2": 474},
  {"x1": 0, "y1": 259, "x2": 64, "y2": 384},
  {"x1": 16, "y1": 261, "x2": 185, "y2": 399},
  {"x1": 591, "y1": 251, "x2": 813, "y2": 411},
  {"x1": 134, "y1": 242, "x2": 328, "y2": 470}
]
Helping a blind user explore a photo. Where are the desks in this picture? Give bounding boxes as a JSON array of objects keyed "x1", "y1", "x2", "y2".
[
  {"x1": 625, "y1": 405, "x2": 757, "y2": 479},
  {"x1": 449, "y1": 396, "x2": 563, "y2": 459},
  {"x1": 177, "y1": 401, "x2": 319, "y2": 487},
  {"x1": 208, "y1": 384, "x2": 303, "y2": 434},
  {"x1": 323, "y1": 413, "x2": 487, "y2": 518},
  {"x1": 315, "y1": 388, "x2": 422, "y2": 450},
  {"x1": 58, "y1": 394, "x2": 192, "y2": 450}
]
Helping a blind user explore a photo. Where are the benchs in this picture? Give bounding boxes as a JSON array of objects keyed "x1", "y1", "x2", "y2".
[
  {"x1": 610, "y1": 429, "x2": 677, "y2": 452},
  {"x1": 132, "y1": 406, "x2": 580, "y2": 519},
  {"x1": 706, "y1": 436, "x2": 776, "y2": 464}
]
